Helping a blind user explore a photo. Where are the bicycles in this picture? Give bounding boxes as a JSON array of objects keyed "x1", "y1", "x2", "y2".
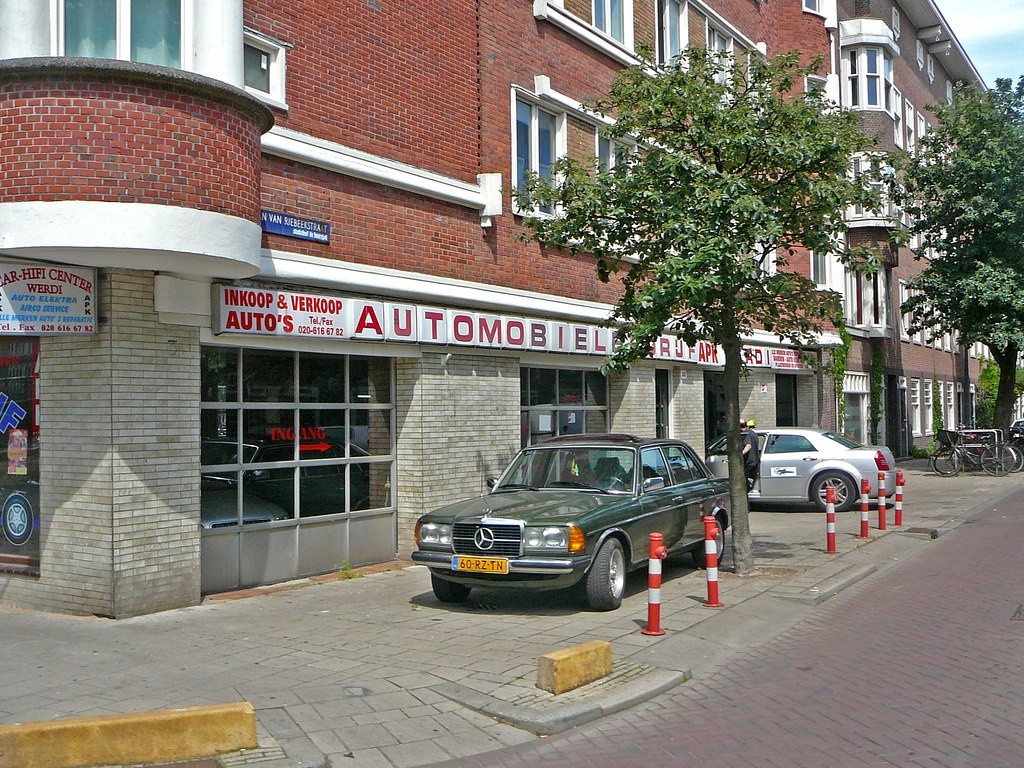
[{"x1": 926, "y1": 427, "x2": 1024, "y2": 476}]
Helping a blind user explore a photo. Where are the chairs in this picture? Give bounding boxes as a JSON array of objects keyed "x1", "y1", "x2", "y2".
[{"x1": 590, "y1": 456, "x2": 628, "y2": 491}]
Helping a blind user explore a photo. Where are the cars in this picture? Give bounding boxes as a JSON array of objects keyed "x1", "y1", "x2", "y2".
[
  {"x1": 408, "y1": 431, "x2": 730, "y2": 612},
  {"x1": 201, "y1": 423, "x2": 371, "y2": 527},
  {"x1": 707, "y1": 425, "x2": 901, "y2": 512}
]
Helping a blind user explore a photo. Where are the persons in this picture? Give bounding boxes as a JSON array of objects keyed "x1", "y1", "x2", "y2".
[{"x1": 739, "y1": 418, "x2": 759, "y2": 513}]
